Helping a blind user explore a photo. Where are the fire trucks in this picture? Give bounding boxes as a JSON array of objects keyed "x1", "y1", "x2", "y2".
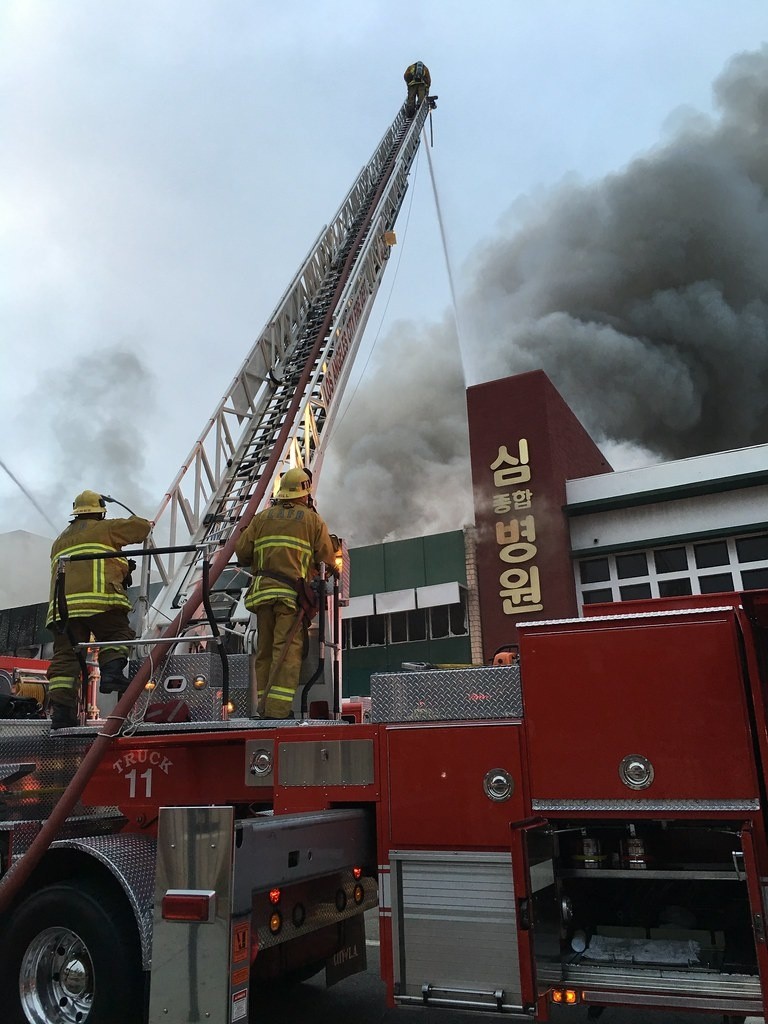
[{"x1": 0, "y1": 61, "x2": 768, "y2": 1024}]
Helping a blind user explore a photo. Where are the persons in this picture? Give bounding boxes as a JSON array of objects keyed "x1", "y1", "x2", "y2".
[
  {"x1": 44, "y1": 490, "x2": 151, "y2": 729},
  {"x1": 236, "y1": 468, "x2": 340, "y2": 720}
]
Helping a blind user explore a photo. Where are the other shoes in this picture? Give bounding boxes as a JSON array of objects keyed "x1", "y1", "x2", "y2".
[{"x1": 257, "y1": 709, "x2": 295, "y2": 719}]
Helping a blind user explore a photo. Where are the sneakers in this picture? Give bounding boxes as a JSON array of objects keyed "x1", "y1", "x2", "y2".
[
  {"x1": 51, "y1": 719, "x2": 79, "y2": 728},
  {"x1": 100, "y1": 669, "x2": 131, "y2": 694}
]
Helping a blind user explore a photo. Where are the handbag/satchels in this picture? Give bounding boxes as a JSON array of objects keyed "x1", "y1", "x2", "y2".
[{"x1": 297, "y1": 577, "x2": 319, "y2": 621}]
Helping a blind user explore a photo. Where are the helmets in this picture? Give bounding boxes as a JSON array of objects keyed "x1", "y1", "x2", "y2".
[
  {"x1": 276, "y1": 468, "x2": 311, "y2": 499},
  {"x1": 68, "y1": 489, "x2": 106, "y2": 516}
]
